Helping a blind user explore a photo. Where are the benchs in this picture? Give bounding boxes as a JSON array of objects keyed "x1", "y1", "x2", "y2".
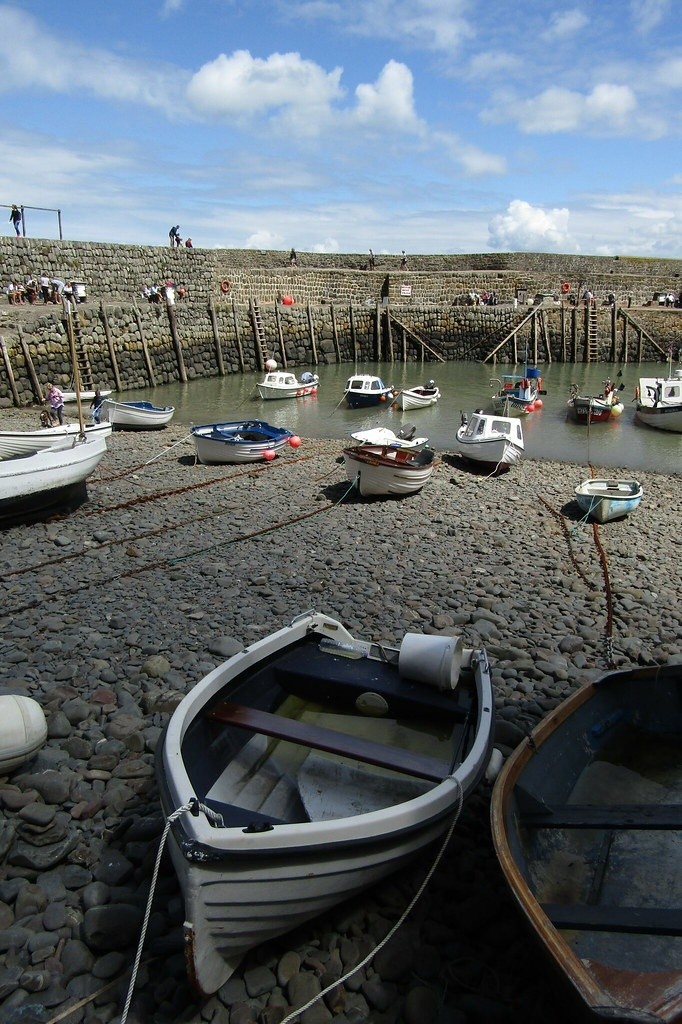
[
  {"x1": 201, "y1": 699, "x2": 450, "y2": 781},
  {"x1": 588, "y1": 489, "x2": 638, "y2": 496},
  {"x1": 537, "y1": 902, "x2": 682, "y2": 936},
  {"x1": 519, "y1": 803, "x2": 682, "y2": 829},
  {"x1": 8, "y1": 292, "x2": 30, "y2": 304}
]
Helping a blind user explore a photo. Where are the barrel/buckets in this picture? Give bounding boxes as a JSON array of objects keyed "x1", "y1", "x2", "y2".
[{"x1": 398, "y1": 633, "x2": 463, "y2": 689}]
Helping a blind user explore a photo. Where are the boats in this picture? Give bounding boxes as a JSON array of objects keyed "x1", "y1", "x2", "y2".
[
  {"x1": 343, "y1": 443, "x2": 435, "y2": 500},
  {"x1": 343, "y1": 373, "x2": 395, "y2": 408},
  {"x1": 570, "y1": 377, "x2": 619, "y2": 424},
  {"x1": 635, "y1": 351, "x2": 682, "y2": 434},
  {"x1": 256, "y1": 371, "x2": 320, "y2": 401},
  {"x1": 0, "y1": 299, "x2": 108, "y2": 531},
  {"x1": 455, "y1": 408, "x2": 525, "y2": 477},
  {"x1": 350, "y1": 422, "x2": 430, "y2": 452},
  {"x1": 0, "y1": 423, "x2": 112, "y2": 461},
  {"x1": 394, "y1": 380, "x2": 441, "y2": 412},
  {"x1": 575, "y1": 479, "x2": 643, "y2": 524},
  {"x1": 61, "y1": 390, "x2": 113, "y2": 403},
  {"x1": 102, "y1": 400, "x2": 175, "y2": 431},
  {"x1": 490, "y1": 663, "x2": 681, "y2": 1024},
  {"x1": 489, "y1": 331, "x2": 540, "y2": 418},
  {"x1": 153, "y1": 606, "x2": 494, "y2": 1005},
  {"x1": 189, "y1": 419, "x2": 292, "y2": 464}
]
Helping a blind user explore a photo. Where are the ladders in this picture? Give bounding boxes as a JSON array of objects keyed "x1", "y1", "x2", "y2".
[
  {"x1": 587, "y1": 299, "x2": 598, "y2": 363},
  {"x1": 248, "y1": 299, "x2": 269, "y2": 372},
  {"x1": 61, "y1": 296, "x2": 95, "y2": 392}
]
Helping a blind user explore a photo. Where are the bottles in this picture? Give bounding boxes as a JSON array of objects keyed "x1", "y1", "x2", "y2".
[{"x1": 319, "y1": 638, "x2": 368, "y2": 659}]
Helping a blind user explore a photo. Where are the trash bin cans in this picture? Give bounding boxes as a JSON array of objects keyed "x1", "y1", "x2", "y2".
[
  {"x1": 517, "y1": 288, "x2": 528, "y2": 302},
  {"x1": 72, "y1": 283, "x2": 87, "y2": 302}
]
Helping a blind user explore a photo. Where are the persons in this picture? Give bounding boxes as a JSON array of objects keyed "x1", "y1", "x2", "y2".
[
  {"x1": 145, "y1": 283, "x2": 166, "y2": 305},
  {"x1": 168, "y1": 225, "x2": 192, "y2": 248},
  {"x1": 7, "y1": 271, "x2": 73, "y2": 306},
  {"x1": 41, "y1": 383, "x2": 64, "y2": 427},
  {"x1": 289, "y1": 248, "x2": 297, "y2": 268},
  {"x1": 8, "y1": 204, "x2": 22, "y2": 238},
  {"x1": 467, "y1": 288, "x2": 675, "y2": 309},
  {"x1": 400, "y1": 250, "x2": 409, "y2": 272},
  {"x1": 368, "y1": 249, "x2": 375, "y2": 271},
  {"x1": 90, "y1": 390, "x2": 108, "y2": 424}
]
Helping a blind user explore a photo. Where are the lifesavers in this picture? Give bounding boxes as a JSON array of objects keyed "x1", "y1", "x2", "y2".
[
  {"x1": 636, "y1": 387, "x2": 639, "y2": 400},
  {"x1": 538, "y1": 378, "x2": 542, "y2": 390},
  {"x1": 222, "y1": 280, "x2": 231, "y2": 291},
  {"x1": 563, "y1": 283, "x2": 571, "y2": 292}
]
